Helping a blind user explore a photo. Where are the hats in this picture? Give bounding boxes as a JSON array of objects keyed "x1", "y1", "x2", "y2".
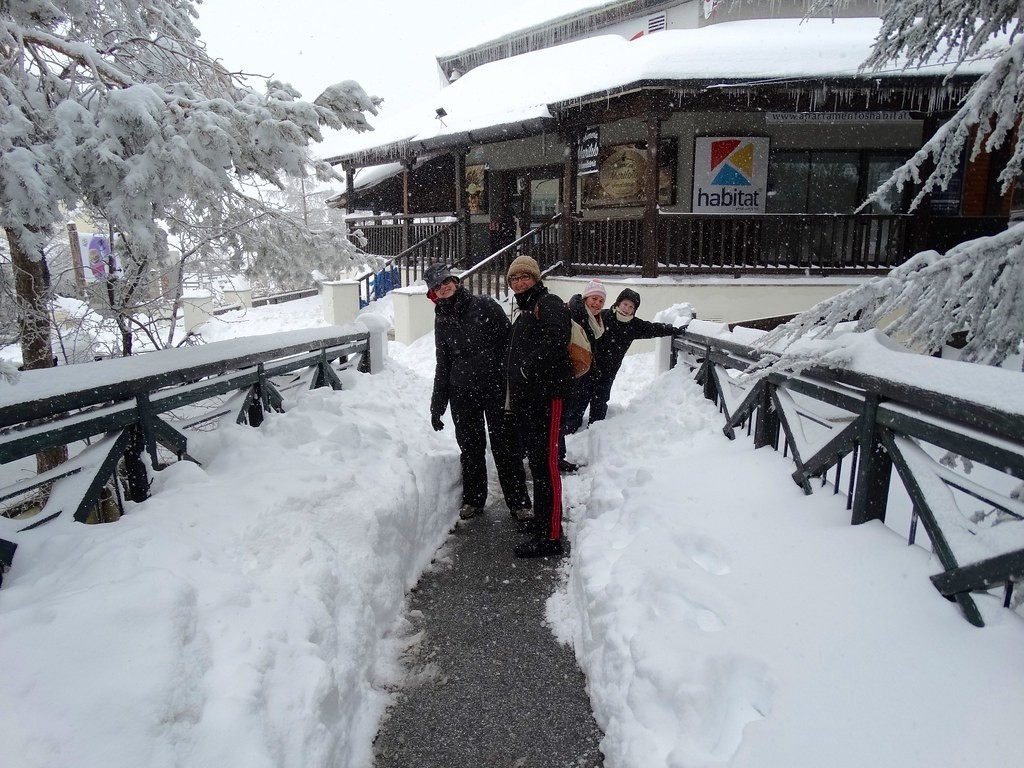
[
  {"x1": 423, "y1": 263, "x2": 460, "y2": 299},
  {"x1": 507, "y1": 255, "x2": 541, "y2": 289},
  {"x1": 582, "y1": 278, "x2": 607, "y2": 301},
  {"x1": 615, "y1": 288, "x2": 641, "y2": 309}
]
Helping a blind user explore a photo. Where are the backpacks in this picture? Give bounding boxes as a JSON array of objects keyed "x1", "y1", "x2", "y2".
[{"x1": 532, "y1": 291, "x2": 593, "y2": 378}]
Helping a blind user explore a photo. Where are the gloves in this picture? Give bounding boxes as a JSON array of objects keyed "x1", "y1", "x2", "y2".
[
  {"x1": 431, "y1": 410, "x2": 445, "y2": 432},
  {"x1": 500, "y1": 405, "x2": 518, "y2": 423},
  {"x1": 677, "y1": 324, "x2": 689, "y2": 336}
]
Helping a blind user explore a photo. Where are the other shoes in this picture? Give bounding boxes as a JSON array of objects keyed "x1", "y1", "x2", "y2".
[
  {"x1": 514, "y1": 531, "x2": 565, "y2": 557},
  {"x1": 458, "y1": 502, "x2": 485, "y2": 519},
  {"x1": 557, "y1": 457, "x2": 586, "y2": 476},
  {"x1": 512, "y1": 508, "x2": 536, "y2": 522}
]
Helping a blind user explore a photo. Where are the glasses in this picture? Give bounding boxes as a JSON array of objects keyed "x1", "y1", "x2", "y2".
[
  {"x1": 433, "y1": 276, "x2": 452, "y2": 291},
  {"x1": 509, "y1": 273, "x2": 532, "y2": 283}
]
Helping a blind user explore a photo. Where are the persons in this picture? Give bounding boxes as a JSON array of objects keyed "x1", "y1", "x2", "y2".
[
  {"x1": 422, "y1": 263, "x2": 534, "y2": 520},
  {"x1": 586, "y1": 288, "x2": 689, "y2": 428},
  {"x1": 504, "y1": 255, "x2": 575, "y2": 557},
  {"x1": 558, "y1": 278, "x2": 607, "y2": 472}
]
